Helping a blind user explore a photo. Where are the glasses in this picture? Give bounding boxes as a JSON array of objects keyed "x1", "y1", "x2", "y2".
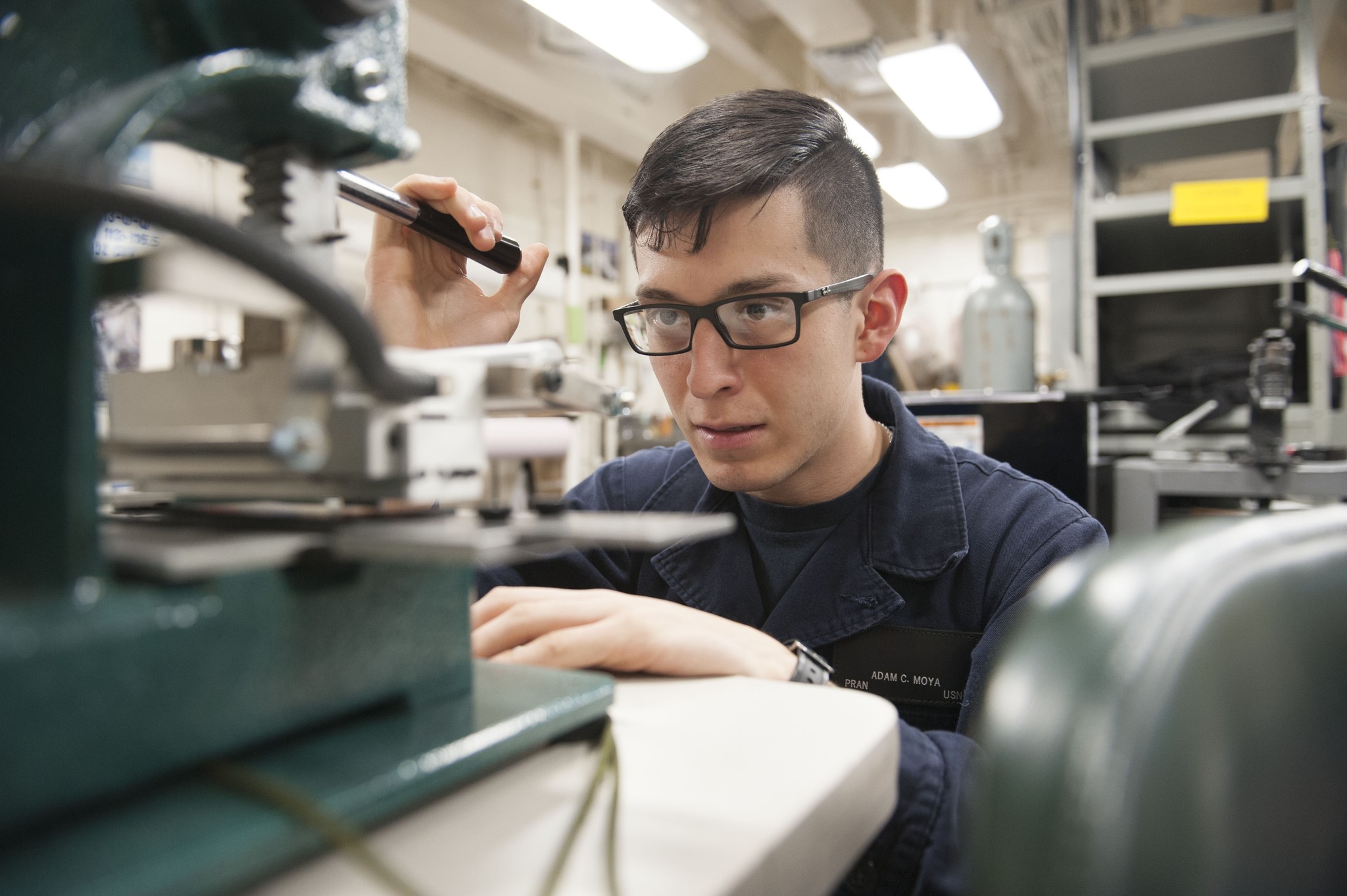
[{"x1": 611, "y1": 272, "x2": 878, "y2": 356}]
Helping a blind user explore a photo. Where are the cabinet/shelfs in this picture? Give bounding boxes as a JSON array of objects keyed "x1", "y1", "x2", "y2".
[{"x1": 1064, "y1": 0, "x2": 1330, "y2": 433}]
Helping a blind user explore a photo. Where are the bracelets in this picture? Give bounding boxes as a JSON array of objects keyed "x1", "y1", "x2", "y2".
[{"x1": 781, "y1": 639, "x2": 836, "y2": 686}]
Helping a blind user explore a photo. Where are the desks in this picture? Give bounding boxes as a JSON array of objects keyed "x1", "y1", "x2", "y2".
[{"x1": 235, "y1": 663, "x2": 904, "y2": 896}]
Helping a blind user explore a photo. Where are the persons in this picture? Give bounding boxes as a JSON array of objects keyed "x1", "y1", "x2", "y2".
[{"x1": 361, "y1": 88, "x2": 1110, "y2": 896}]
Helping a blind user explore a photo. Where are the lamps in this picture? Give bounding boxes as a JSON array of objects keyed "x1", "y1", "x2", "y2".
[
  {"x1": 521, "y1": 0, "x2": 714, "y2": 75},
  {"x1": 875, "y1": 30, "x2": 1007, "y2": 143},
  {"x1": 814, "y1": 89, "x2": 884, "y2": 163},
  {"x1": 874, "y1": 157, "x2": 949, "y2": 211}
]
